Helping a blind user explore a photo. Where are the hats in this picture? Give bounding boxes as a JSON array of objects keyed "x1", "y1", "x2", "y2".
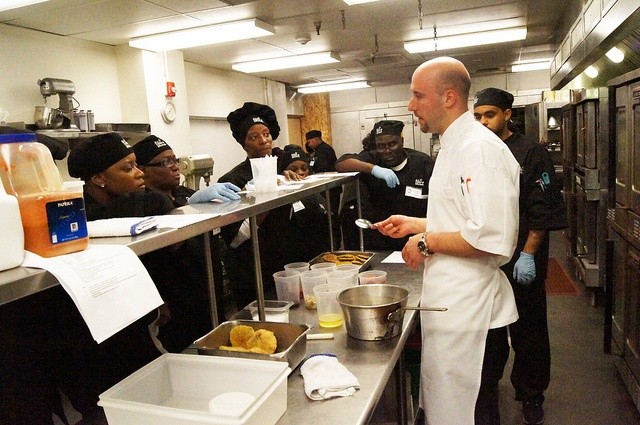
[
  {"x1": 227, "y1": 102, "x2": 280, "y2": 147},
  {"x1": 306, "y1": 130, "x2": 321, "y2": 140},
  {"x1": 374, "y1": 120, "x2": 405, "y2": 136},
  {"x1": 132, "y1": 135, "x2": 172, "y2": 165},
  {"x1": 281, "y1": 144, "x2": 310, "y2": 168},
  {"x1": 474, "y1": 88, "x2": 514, "y2": 109},
  {"x1": 67, "y1": 132, "x2": 134, "y2": 181}
]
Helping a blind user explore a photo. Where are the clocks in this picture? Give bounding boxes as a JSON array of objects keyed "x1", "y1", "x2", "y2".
[{"x1": 164, "y1": 100, "x2": 177, "y2": 122}]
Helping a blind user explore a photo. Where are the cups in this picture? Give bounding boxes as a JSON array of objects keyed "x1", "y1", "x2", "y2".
[
  {"x1": 358, "y1": 270, "x2": 387, "y2": 284},
  {"x1": 332, "y1": 264, "x2": 360, "y2": 286},
  {"x1": 310, "y1": 262, "x2": 337, "y2": 273},
  {"x1": 326, "y1": 270, "x2": 355, "y2": 290},
  {"x1": 313, "y1": 283, "x2": 345, "y2": 327},
  {"x1": 272, "y1": 270, "x2": 300, "y2": 309},
  {"x1": 300, "y1": 270, "x2": 327, "y2": 312},
  {"x1": 249, "y1": 154, "x2": 278, "y2": 196},
  {"x1": 284, "y1": 262, "x2": 311, "y2": 303}
]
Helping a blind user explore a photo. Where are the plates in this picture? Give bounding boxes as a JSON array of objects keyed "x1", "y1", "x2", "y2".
[{"x1": 244, "y1": 299, "x2": 295, "y2": 312}]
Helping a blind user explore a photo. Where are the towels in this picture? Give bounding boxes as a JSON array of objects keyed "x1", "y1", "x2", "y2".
[
  {"x1": 86, "y1": 216, "x2": 160, "y2": 238},
  {"x1": 301, "y1": 351, "x2": 362, "y2": 402}
]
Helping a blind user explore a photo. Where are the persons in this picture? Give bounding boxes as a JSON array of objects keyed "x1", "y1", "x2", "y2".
[
  {"x1": 218, "y1": 103, "x2": 309, "y2": 319},
  {"x1": 306, "y1": 130, "x2": 342, "y2": 251},
  {"x1": 67, "y1": 132, "x2": 145, "y2": 216},
  {"x1": 369, "y1": 56, "x2": 522, "y2": 424},
  {"x1": 280, "y1": 144, "x2": 309, "y2": 181},
  {"x1": 124, "y1": 135, "x2": 242, "y2": 208},
  {"x1": 474, "y1": 87, "x2": 568, "y2": 424},
  {"x1": 304, "y1": 141, "x2": 317, "y2": 174},
  {"x1": 335, "y1": 119, "x2": 435, "y2": 258}
]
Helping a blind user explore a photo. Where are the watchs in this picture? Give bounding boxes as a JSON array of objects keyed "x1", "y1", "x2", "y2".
[{"x1": 417, "y1": 240, "x2": 426, "y2": 252}]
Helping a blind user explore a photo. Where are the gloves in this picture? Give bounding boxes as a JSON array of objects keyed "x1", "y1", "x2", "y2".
[
  {"x1": 370, "y1": 165, "x2": 400, "y2": 188},
  {"x1": 513, "y1": 252, "x2": 536, "y2": 285},
  {"x1": 188, "y1": 181, "x2": 241, "y2": 204}
]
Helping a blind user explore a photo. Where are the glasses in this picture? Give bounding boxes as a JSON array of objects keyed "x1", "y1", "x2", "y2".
[{"x1": 144, "y1": 158, "x2": 181, "y2": 168}]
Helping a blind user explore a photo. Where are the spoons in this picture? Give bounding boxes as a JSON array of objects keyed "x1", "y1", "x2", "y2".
[{"x1": 355, "y1": 218, "x2": 372, "y2": 230}]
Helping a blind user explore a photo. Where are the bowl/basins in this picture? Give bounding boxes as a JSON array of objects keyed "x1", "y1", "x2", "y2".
[{"x1": 208, "y1": 391, "x2": 255, "y2": 417}]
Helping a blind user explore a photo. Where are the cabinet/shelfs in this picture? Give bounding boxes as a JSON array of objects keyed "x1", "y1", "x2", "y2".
[
  {"x1": 613, "y1": 81, "x2": 640, "y2": 381},
  {"x1": 542, "y1": 102, "x2": 562, "y2": 169},
  {"x1": 575, "y1": 98, "x2": 603, "y2": 266}
]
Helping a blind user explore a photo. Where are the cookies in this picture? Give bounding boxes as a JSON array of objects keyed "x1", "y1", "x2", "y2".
[{"x1": 323, "y1": 253, "x2": 367, "y2": 265}]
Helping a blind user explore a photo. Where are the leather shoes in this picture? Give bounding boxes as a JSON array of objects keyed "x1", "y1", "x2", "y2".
[{"x1": 522, "y1": 398, "x2": 545, "y2": 423}]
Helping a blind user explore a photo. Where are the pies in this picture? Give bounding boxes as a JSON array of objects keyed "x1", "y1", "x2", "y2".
[{"x1": 219, "y1": 326, "x2": 274, "y2": 355}]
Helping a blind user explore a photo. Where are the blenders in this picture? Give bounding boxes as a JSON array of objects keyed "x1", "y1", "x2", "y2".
[{"x1": 33, "y1": 77, "x2": 80, "y2": 140}]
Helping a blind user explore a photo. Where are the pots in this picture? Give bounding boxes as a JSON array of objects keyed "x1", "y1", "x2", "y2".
[{"x1": 336, "y1": 283, "x2": 447, "y2": 341}]
